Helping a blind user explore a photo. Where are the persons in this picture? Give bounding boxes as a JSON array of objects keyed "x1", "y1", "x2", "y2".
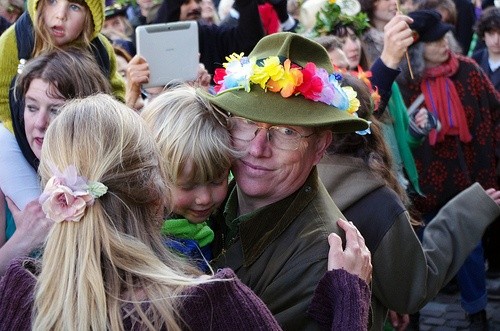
[
  {"x1": 1, "y1": 92, "x2": 372, "y2": 331},
  {"x1": 211, "y1": 32, "x2": 349, "y2": 331},
  {"x1": 1, "y1": 1, "x2": 126, "y2": 136},
  {"x1": 0, "y1": 47, "x2": 116, "y2": 276},
  {"x1": 0, "y1": 0, "x2": 500, "y2": 204},
  {"x1": 315, "y1": 73, "x2": 499, "y2": 330},
  {"x1": 139, "y1": 82, "x2": 247, "y2": 277}
]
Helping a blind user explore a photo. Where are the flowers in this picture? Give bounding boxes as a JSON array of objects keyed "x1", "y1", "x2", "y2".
[
  {"x1": 309, "y1": 0, "x2": 372, "y2": 40},
  {"x1": 39, "y1": 164, "x2": 109, "y2": 223},
  {"x1": 213, "y1": 53, "x2": 371, "y2": 136}
]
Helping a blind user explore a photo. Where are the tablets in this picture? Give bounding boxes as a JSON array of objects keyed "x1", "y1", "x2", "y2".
[{"x1": 136, "y1": 20, "x2": 199, "y2": 88}]
